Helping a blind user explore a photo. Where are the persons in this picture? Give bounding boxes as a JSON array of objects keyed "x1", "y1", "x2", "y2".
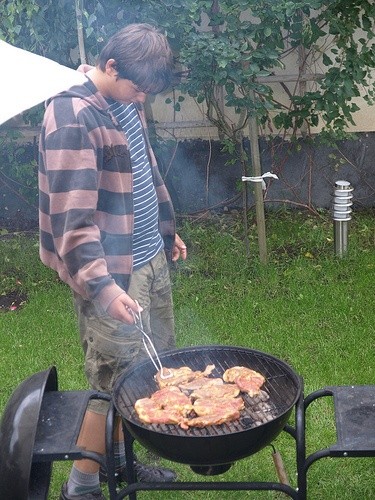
[{"x1": 38, "y1": 22, "x2": 186, "y2": 500}]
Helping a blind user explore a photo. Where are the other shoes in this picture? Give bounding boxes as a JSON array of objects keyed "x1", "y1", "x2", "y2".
[
  {"x1": 59, "y1": 480, "x2": 107, "y2": 499},
  {"x1": 99, "y1": 455, "x2": 177, "y2": 486}
]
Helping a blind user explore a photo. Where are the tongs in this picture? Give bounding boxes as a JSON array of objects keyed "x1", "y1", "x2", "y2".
[{"x1": 127, "y1": 300, "x2": 174, "y2": 383}]
